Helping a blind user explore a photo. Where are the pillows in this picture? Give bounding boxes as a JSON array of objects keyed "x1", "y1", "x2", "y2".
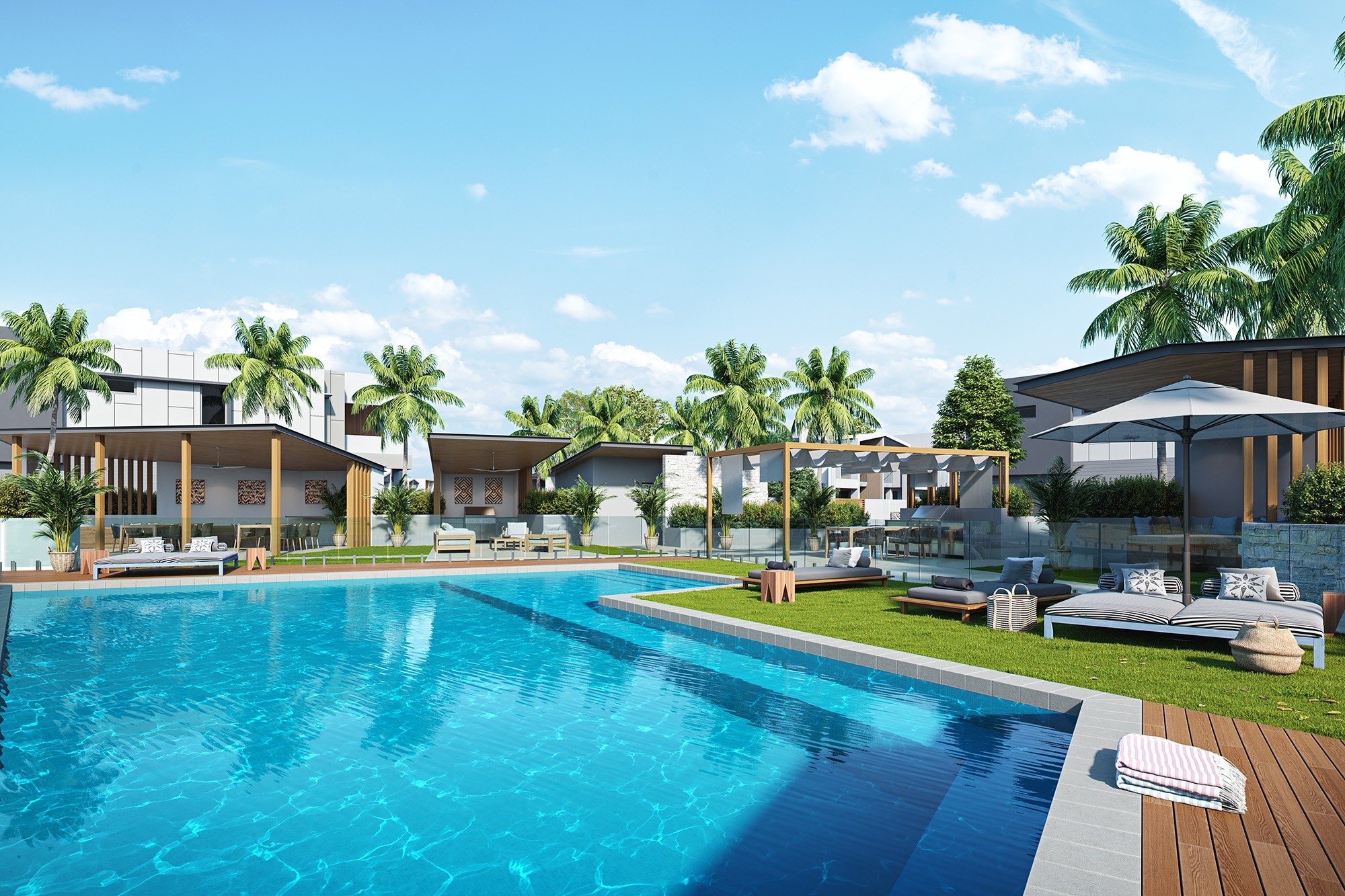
[
  {"x1": 827, "y1": 547, "x2": 1286, "y2": 601},
  {"x1": 1133, "y1": 516, "x2": 1238, "y2": 535},
  {"x1": 189, "y1": 540, "x2": 214, "y2": 553},
  {"x1": 141, "y1": 540, "x2": 165, "y2": 552},
  {"x1": 191, "y1": 536, "x2": 219, "y2": 552},
  {"x1": 133, "y1": 536, "x2": 163, "y2": 552}
]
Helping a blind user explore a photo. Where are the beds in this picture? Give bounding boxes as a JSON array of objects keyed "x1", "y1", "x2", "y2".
[
  {"x1": 91, "y1": 537, "x2": 240, "y2": 579},
  {"x1": 735, "y1": 555, "x2": 1326, "y2": 670}
]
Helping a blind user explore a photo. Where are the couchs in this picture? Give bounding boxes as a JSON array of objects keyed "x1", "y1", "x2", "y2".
[
  {"x1": 1128, "y1": 535, "x2": 1242, "y2": 553},
  {"x1": 434, "y1": 522, "x2": 564, "y2": 554}
]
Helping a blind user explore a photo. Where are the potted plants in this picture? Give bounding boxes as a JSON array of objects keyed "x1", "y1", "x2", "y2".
[
  {"x1": 1019, "y1": 455, "x2": 1106, "y2": 569},
  {"x1": 564, "y1": 472, "x2": 841, "y2": 551},
  {"x1": 314, "y1": 474, "x2": 430, "y2": 547},
  {"x1": 12, "y1": 450, "x2": 119, "y2": 573}
]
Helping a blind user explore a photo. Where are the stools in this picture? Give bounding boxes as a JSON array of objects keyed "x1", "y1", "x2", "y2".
[{"x1": 761, "y1": 570, "x2": 796, "y2": 603}]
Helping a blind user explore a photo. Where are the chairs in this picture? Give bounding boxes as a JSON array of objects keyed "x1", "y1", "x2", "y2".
[
  {"x1": 827, "y1": 525, "x2": 935, "y2": 561},
  {"x1": 108, "y1": 522, "x2": 322, "y2": 552}
]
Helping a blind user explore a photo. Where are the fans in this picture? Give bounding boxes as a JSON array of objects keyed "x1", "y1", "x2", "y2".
[
  {"x1": 468, "y1": 452, "x2": 520, "y2": 472},
  {"x1": 191, "y1": 446, "x2": 247, "y2": 470}
]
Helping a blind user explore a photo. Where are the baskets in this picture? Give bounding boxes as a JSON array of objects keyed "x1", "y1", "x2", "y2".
[{"x1": 986, "y1": 583, "x2": 1038, "y2": 632}]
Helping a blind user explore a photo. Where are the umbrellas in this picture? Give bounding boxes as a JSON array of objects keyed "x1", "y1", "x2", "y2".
[{"x1": 1028, "y1": 375, "x2": 1345, "y2": 607}]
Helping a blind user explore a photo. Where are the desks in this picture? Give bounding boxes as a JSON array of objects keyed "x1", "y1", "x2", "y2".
[
  {"x1": 80, "y1": 550, "x2": 109, "y2": 574},
  {"x1": 119, "y1": 525, "x2": 185, "y2": 552},
  {"x1": 489, "y1": 536, "x2": 523, "y2": 551},
  {"x1": 524, "y1": 534, "x2": 569, "y2": 555},
  {"x1": 825, "y1": 526, "x2": 928, "y2": 560},
  {"x1": 246, "y1": 547, "x2": 267, "y2": 571},
  {"x1": 236, "y1": 525, "x2": 296, "y2": 551}
]
